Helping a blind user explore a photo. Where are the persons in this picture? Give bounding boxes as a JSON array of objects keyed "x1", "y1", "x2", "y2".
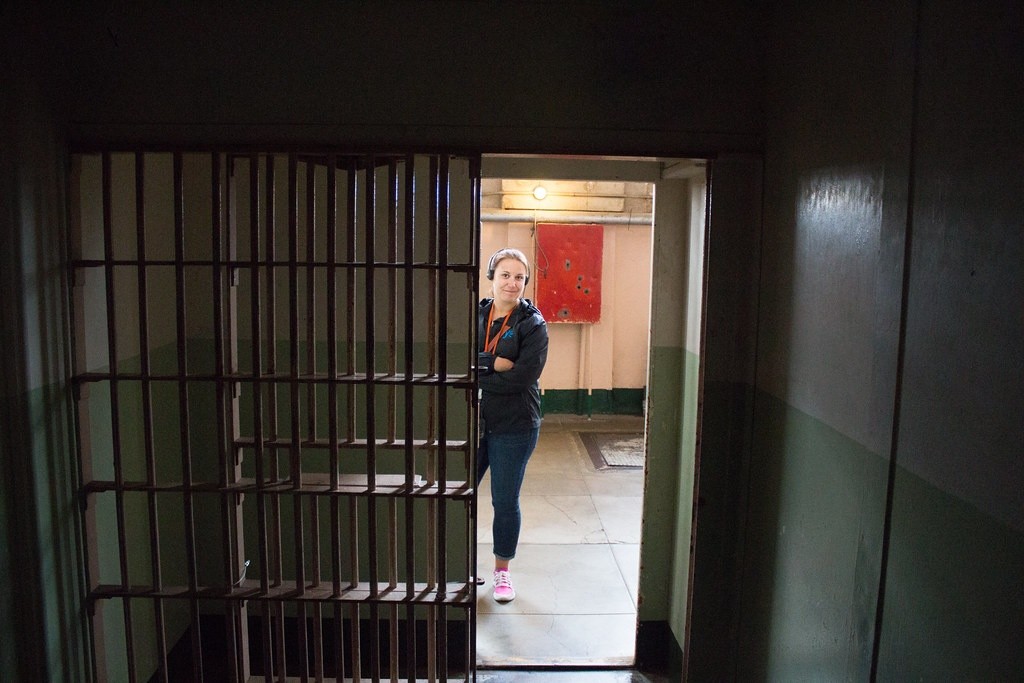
[{"x1": 476, "y1": 248, "x2": 548, "y2": 602}]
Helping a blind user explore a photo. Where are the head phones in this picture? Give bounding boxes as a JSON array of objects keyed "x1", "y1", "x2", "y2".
[{"x1": 487, "y1": 248, "x2": 530, "y2": 285}]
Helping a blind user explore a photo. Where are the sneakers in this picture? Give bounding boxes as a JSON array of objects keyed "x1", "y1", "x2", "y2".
[{"x1": 493, "y1": 567, "x2": 515, "y2": 601}]
